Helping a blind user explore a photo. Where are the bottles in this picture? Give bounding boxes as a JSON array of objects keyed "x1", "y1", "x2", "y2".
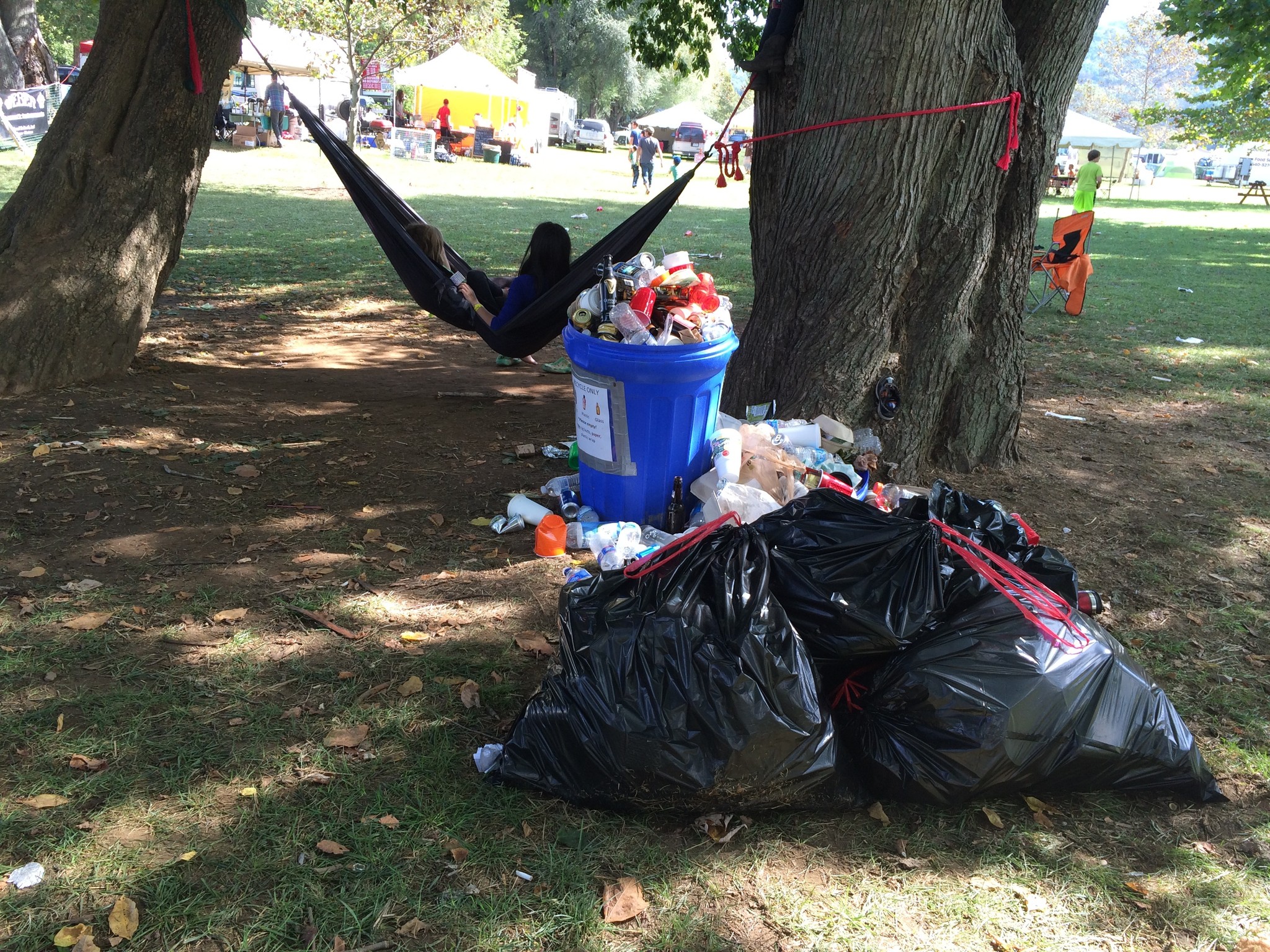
[
  {"x1": 779, "y1": 476, "x2": 809, "y2": 499},
  {"x1": 872, "y1": 482, "x2": 922, "y2": 504},
  {"x1": 540, "y1": 473, "x2": 581, "y2": 497},
  {"x1": 784, "y1": 445, "x2": 833, "y2": 467},
  {"x1": 593, "y1": 253, "x2": 730, "y2": 346},
  {"x1": 564, "y1": 505, "x2": 642, "y2": 584},
  {"x1": 665, "y1": 475, "x2": 685, "y2": 535},
  {"x1": 596, "y1": 403, "x2": 600, "y2": 415},
  {"x1": 761, "y1": 419, "x2": 807, "y2": 435},
  {"x1": 851, "y1": 428, "x2": 883, "y2": 457},
  {"x1": 582, "y1": 395, "x2": 587, "y2": 410},
  {"x1": 689, "y1": 500, "x2": 706, "y2": 528}
]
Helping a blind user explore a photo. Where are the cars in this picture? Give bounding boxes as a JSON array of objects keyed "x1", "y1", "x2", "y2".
[{"x1": 614, "y1": 127, "x2": 636, "y2": 146}]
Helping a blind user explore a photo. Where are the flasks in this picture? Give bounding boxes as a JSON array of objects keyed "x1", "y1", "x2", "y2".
[{"x1": 1076, "y1": 590, "x2": 1102, "y2": 617}]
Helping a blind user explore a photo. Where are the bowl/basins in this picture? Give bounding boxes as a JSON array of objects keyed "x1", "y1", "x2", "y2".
[{"x1": 854, "y1": 469, "x2": 870, "y2": 501}]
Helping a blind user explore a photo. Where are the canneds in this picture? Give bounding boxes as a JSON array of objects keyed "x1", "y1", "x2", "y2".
[
  {"x1": 801, "y1": 473, "x2": 821, "y2": 489},
  {"x1": 618, "y1": 285, "x2": 690, "y2": 306},
  {"x1": 770, "y1": 433, "x2": 794, "y2": 452},
  {"x1": 559, "y1": 490, "x2": 580, "y2": 518},
  {"x1": 571, "y1": 308, "x2": 620, "y2": 343},
  {"x1": 488, "y1": 513, "x2": 524, "y2": 534},
  {"x1": 627, "y1": 544, "x2": 661, "y2": 566},
  {"x1": 625, "y1": 252, "x2": 655, "y2": 271},
  {"x1": 686, "y1": 311, "x2": 718, "y2": 330}
]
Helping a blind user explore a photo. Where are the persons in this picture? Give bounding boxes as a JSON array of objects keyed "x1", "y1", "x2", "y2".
[
  {"x1": 407, "y1": 222, "x2": 572, "y2": 364},
  {"x1": 1046, "y1": 149, "x2": 1103, "y2": 215},
  {"x1": 262, "y1": 72, "x2": 752, "y2": 196}
]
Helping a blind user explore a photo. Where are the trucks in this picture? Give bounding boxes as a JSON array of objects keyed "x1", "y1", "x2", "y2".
[
  {"x1": 533, "y1": 88, "x2": 578, "y2": 146},
  {"x1": 727, "y1": 133, "x2": 748, "y2": 149}
]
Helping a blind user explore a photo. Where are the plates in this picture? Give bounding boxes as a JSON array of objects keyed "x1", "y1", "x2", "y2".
[{"x1": 875, "y1": 494, "x2": 893, "y2": 511}]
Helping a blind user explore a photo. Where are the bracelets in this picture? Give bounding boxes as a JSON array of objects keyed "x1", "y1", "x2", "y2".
[{"x1": 474, "y1": 304, "x2": 484, "y2": 311}]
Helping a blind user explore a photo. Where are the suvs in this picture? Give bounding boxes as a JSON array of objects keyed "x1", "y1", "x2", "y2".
[
  {"x1": 579, "y1": 120, "x2": 615, "y2": 153},
  {"x1": 54, "y1": 64, "x2": 82, "y2": 85}
]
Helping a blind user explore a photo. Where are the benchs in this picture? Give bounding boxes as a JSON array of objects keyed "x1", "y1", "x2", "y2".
[
  {"x1": 1238, "y1": 193, "x2": 1246, "y2": 196},
  {"x1": 1264, "y1": 195, "x2": 1270, "y2": 197}
]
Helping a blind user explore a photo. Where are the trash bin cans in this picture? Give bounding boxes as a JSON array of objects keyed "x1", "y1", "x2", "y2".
[
  {"x1": 561, "y1": 319, "x2": 739, "y2": 524},
  {"x1": 480, "y1": 143, "x2": 501, "y2": 165},
  {"x1": 485, "y1": 139, "x2": 516, "y2": 164}
]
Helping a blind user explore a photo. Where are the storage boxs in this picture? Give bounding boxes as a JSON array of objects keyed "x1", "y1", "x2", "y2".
[{"x1": 232, "y1": 121, "x2": 273, "y2": 148}]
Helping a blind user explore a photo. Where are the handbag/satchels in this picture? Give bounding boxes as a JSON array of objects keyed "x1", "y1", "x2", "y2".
[{"x1": 493, "y1": 478, "x2": 1218, "y2": 815}]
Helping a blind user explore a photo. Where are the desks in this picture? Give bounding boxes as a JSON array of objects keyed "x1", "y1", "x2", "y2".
[
  {"x1": 1238, "y1": 182, "x2": 1270, "y2": 206},
  {"x1": 227, "y1": 113, "x2": 288, "y2": 147},
  {"x1": 1045, "y1": 177, "x2": 1076, "y2": 197},
  {"x1": 488, "y1": 139, "x2": 514, "y2": 164}
]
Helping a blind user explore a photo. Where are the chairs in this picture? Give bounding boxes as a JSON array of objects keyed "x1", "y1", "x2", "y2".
[
  {"x1": 213, "y1": 104, "x2": 236, "y2": 143},
  {"x1": 1048, "y1": 177, "x2": 1076, "y2": 197},
  {"x1": 1025, "y1": 208, "x2": 1095, "y2": 316},
  {"x1": 1101, "y1": 179, "x2": 1117, "y2": 199}
]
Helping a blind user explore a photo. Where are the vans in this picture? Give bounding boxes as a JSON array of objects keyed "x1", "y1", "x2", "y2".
[{"x1": 672, "y1": 121, "x2": 707, "y2": 159}]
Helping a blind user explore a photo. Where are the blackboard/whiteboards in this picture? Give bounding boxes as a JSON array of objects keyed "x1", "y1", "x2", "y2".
[{"x1": 473, "y1": 127, "x2": 495, "y2": 159}]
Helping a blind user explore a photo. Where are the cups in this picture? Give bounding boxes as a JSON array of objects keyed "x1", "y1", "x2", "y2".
[
  {"x1": 534, "y1": 515, "x2": 567, "y2": 557},
  {"x1": 507, "y1": 493, "x2": 553, "y2": 526},
  {"x1": 817, "y1": 469, "x2": 853, "y2": 496},
  {"x1": 709, "y1": 428, "x2": 742, "y2": 483},
  {"x1": 568, "y1": 441, "x2": 580, "y2": 471},
  {"x1": 739, "y1": 419, "x2": 752, "y2": 426},
  {"x1": 639, "y1": 525, "x2": 678, "y2": 548},
  {"x1": 778, "y1": 424, "x2": 821, "y2": 448},
  {"x1": 1010, "y1": 512, "x2": 1039, "y2": 547},
  {"x1": 580, "y1": 291, "x2": 601, "y2": 315}
]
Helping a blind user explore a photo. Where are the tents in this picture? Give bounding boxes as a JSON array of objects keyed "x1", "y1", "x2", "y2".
[
  {"x1": 1058, "y1": 109, "x2": 1143, "y2": 200},
  {"x1": 78, "y1": 17, "x2": 756, "y2": 162}
]
